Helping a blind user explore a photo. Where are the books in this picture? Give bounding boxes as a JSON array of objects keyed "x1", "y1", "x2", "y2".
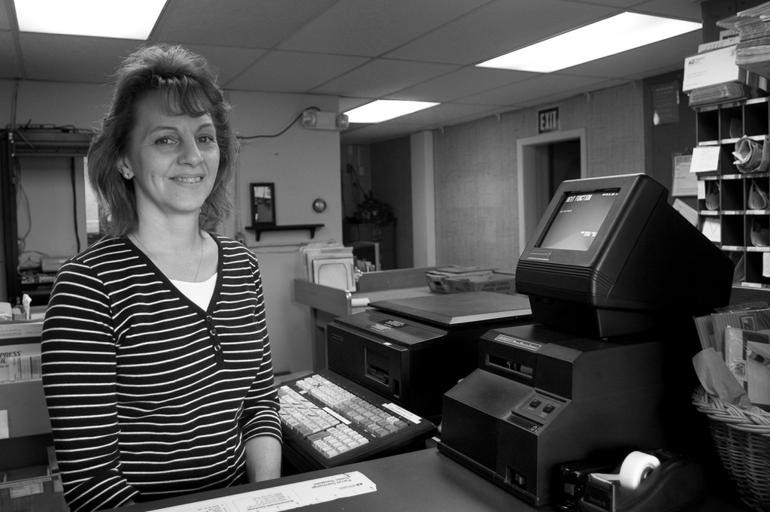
[
  {"x1": 299, "y1": 239, "x2": 360, "y2": 297},
  {"x1": 678, "y1": 0, "x2": 770, "y2": 112},
  {"x1": 692, "y1": 304, "x2": 769, "y2": 407}
]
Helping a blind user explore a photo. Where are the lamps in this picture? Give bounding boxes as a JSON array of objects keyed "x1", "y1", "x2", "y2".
[{"x1": 303, "y1": 110, "x2": 349, "y2": 131}]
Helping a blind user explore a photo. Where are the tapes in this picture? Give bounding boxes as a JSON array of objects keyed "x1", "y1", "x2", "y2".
[{"x1": 619, "y1": 451, "x2": 660, "y2": 490}]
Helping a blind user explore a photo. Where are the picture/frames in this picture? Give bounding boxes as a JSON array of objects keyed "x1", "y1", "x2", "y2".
[{"x1": 250, "y1": 181, "x2": 277, "y2": 227}]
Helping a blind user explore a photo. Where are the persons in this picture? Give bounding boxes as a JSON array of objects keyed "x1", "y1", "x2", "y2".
[{"x1": 38, "y1": 44, "x2": 286, "y2": 510}]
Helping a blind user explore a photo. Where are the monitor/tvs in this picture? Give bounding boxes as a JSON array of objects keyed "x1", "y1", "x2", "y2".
[{"x1": 515, "y1": 173, "x2": 734, "y2": 342}]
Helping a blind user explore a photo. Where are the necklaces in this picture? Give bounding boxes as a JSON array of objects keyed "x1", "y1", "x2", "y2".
[{"x1": 132, "y1": 233, "x2": 205, "y2": 285}]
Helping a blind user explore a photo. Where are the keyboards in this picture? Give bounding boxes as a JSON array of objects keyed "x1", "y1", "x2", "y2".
[{"x1": 259, "y1": 367, "x2": 437, "y2": 469}]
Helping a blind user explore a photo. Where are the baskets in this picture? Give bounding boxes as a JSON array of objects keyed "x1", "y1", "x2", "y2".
[{"x1": 692, "y1": 386, "x2": 770, "y2": 512}]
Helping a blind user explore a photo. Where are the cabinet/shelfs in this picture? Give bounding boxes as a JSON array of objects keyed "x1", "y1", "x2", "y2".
[{"x1": 691, "y1": 96, "x2": 769, "y2": 310}]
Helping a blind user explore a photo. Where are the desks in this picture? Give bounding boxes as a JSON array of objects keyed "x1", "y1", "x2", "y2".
[{"x1": 111, "y1": 446, "x2": 538, "y2": 512}]
